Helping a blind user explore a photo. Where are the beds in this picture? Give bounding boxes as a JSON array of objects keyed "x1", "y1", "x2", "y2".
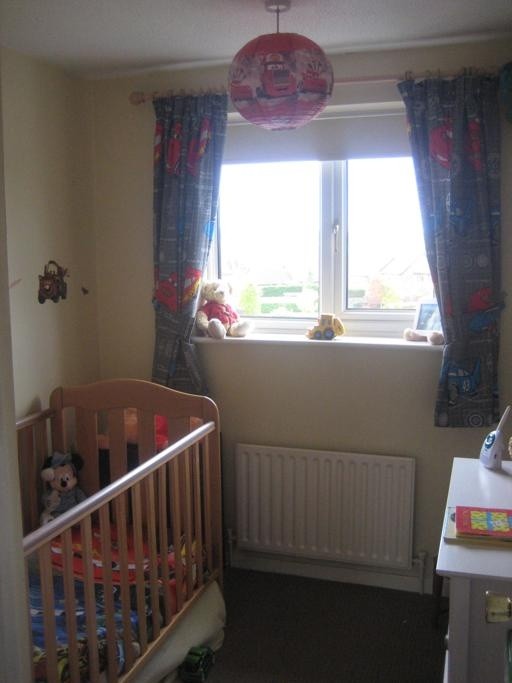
[{"x1": 15, "y1": 377, "x2": 225, "y2": 682}]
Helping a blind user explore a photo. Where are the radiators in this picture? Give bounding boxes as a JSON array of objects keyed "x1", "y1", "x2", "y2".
[{"x1": 233, "y1": 442, "x2": 416, "y2": 571}]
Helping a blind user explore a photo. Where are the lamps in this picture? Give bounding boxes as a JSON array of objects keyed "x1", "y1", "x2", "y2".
[{"x1": 227, "y1": 0, "x2": 334, "y2": 133}]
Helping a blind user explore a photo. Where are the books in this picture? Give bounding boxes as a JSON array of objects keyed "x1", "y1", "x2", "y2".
[{"x1": 443, "y1": 506, "x2": 512, "y2": 547}]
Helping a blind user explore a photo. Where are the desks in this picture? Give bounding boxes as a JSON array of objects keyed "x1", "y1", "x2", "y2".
[{"x1": 434, "y1": 456, "x2": 512, "y2": 682}]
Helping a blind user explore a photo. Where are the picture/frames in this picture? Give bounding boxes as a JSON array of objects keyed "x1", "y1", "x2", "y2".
[{"x1": 411, "y1": 298, "x2": 443, "y2": 337}]
